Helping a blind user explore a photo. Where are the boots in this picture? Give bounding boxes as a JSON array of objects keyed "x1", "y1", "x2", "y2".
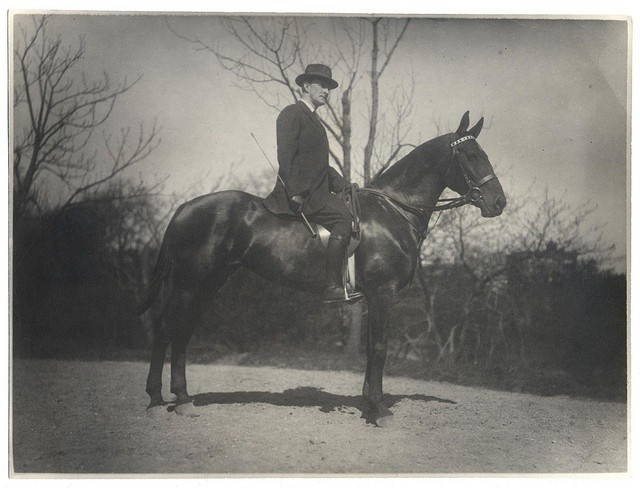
[{"x1": 319, "y1": 234, "x2": 365, "y2": 307}]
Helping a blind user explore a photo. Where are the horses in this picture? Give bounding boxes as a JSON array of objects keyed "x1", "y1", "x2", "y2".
[{"x1": 145, "y1": 109, "x2": 504, "y2": 426}]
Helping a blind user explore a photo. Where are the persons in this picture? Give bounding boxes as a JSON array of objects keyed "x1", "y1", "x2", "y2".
[{"x1": 270, "y1": 65, "x2": 363, "y2": 301}]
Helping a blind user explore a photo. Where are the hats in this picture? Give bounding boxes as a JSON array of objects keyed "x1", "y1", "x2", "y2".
[{"x1": 295, "y1": 64, "x2": 340, "y2": 91}]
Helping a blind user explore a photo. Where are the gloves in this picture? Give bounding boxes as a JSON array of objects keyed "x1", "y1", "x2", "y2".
[
  {"x1": 289, "y1": 193, "x2": 307, "y2": 216},
  {"x1": 352, "y1": 183, "x2": 360, "y2": 194}
]
